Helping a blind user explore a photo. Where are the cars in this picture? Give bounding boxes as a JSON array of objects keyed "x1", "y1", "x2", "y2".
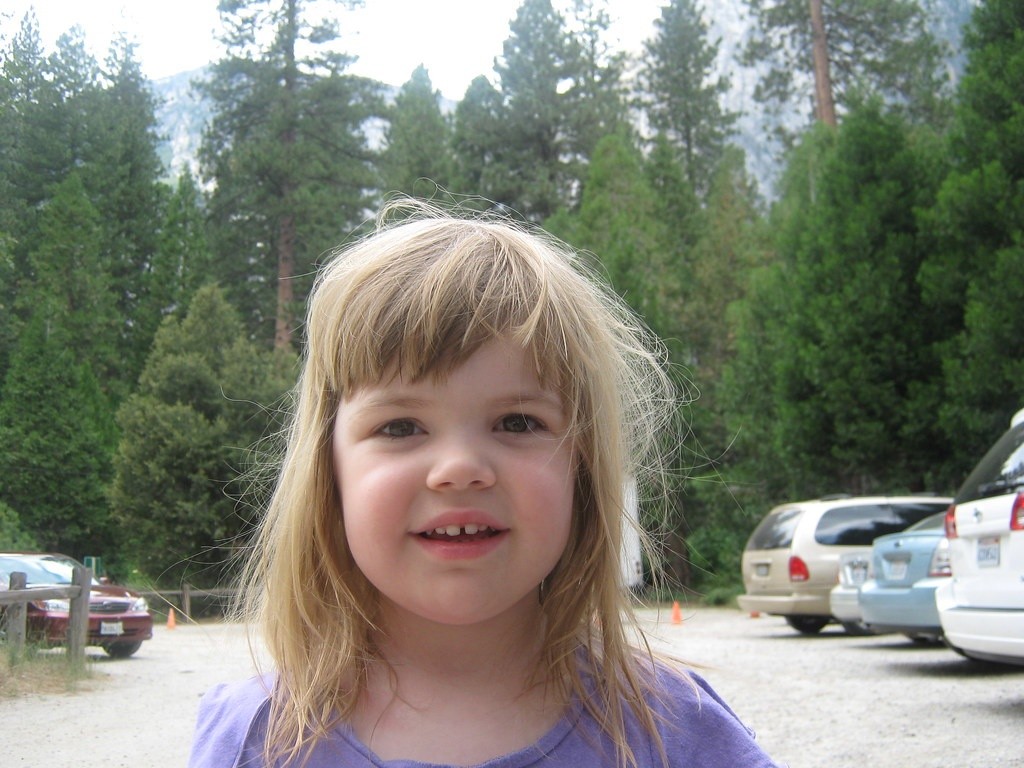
[
  {"x1": 828, "y1": 550, "x2": 896, "y2": 639},
  {"x1": 0, "y1": 550, "x2": 152, "y2": 659},
  {"x1": 860, "y1": 510, "x2": 945, "y2": 646}
]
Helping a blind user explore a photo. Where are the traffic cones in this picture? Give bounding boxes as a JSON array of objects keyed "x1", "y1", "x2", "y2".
[
  {"x1": 164, "y1": 607, "x2": 177, "y2": 629},
  {"x1": 748, "y1": 612, "x2": 760, "y2": 618},
  {"x1": 669, "y1": 600, "x2": 683, "y2": 625}
]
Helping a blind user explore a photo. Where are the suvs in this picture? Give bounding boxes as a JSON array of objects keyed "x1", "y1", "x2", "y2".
[
  {"x1": 934, "y1": 403, "x2": 1024, "y2": 668},
  {"x1": 734, "y1": 490, "x2": 954, "y2": 636}
]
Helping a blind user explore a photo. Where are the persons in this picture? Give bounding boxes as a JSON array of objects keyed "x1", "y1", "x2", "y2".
[{"x1": 188, "y1": 213, "x2": 780, "y2": 768}]
